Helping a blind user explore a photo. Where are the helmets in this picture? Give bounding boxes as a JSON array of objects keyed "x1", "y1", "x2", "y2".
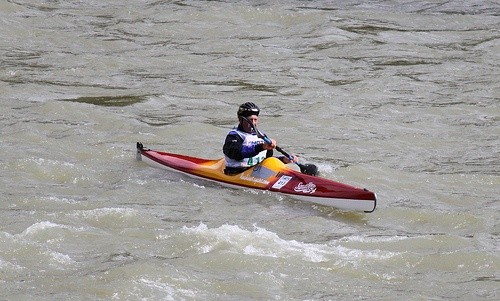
[{"x1": 237, "y1": 102, "x2": 260, "y2": 118}]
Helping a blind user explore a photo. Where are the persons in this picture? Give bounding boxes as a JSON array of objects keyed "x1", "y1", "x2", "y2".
[{"x1": 221, "y1": 102, "x2": 298, "y2": 176}]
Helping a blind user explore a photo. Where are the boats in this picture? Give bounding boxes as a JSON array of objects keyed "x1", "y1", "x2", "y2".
[{"x1": 135, "y1": 140, "x2": 377, "y2": 213}]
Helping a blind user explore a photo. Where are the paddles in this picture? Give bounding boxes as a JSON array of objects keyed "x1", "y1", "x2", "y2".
[{"x1": 244, "y1": 116, "x2": 318, "y2": 176}]
